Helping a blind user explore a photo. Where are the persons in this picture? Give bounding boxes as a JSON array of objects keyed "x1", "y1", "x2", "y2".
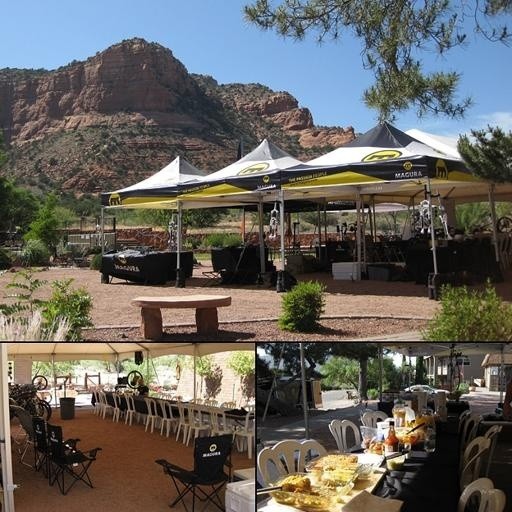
[
  {"x1": 503, "y1": 365, "x2": 512, "y2": 421},
  {"x1": 136, "y1": 386, "x2": 149, "y2": 401}
]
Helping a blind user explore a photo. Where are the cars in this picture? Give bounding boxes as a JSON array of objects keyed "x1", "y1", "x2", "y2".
[
  {"x1": 402, "y1": 384, "x2": 449, "y2": 396},
  {"x1": 151, "y1": 377, "x2": 178, "y2": 393}
]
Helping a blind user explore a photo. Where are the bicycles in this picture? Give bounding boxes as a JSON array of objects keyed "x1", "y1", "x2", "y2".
[{"x1": 10, "y1": 375, "x2": 53, "y2": 419}]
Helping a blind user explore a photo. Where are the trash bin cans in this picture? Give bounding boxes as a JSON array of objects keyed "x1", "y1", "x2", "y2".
[
  {"x1": 378, "y1": 401, "x2": 395, "y2": 422},
  {"x1": 314, "y1": 246, "x2": 336, "y2": 261},
  {"x1": 60, "y1": 398, "x2": 75, "y2": 420}
]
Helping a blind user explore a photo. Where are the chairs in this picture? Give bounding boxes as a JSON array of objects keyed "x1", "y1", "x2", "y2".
[
  {"x1": 8, "y1": 371, "x2": 254, "y2": 512},
  {"x1": 255, "y1": 384, "x2": 512, "y2": 512}
]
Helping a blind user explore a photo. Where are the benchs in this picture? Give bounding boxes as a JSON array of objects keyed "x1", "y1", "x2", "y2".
[{"x1": 130, "y1": 294, "x2": 233, "y2": 340}]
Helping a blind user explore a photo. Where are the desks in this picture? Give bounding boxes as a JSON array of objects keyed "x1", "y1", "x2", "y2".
[{"x1": 93, "y1": 227, "x2": 512, "y2": 291}]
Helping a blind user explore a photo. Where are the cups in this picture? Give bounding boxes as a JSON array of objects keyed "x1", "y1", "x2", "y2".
[
  {"x1": 420, "y1": 407, "x2": 433, "y2": 418},
  {"x1": 392, "y1": 403, "x2": 405, "y2": 425}
]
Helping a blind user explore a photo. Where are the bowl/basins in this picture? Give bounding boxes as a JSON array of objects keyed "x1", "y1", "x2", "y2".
[
  {"x1": 269, "y1": 452, "x2": 372, "y2": 508},
  {"x1": 395, "y1": 427, "x2": 426, "y2": 443}
]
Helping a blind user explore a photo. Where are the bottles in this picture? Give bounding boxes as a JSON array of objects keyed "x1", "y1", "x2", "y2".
[
  {"x1": 424, "y1": 424, "x2": 436, "y2": 452},
  {"x1": 384, "y1": 421, "x2": 398, "y2": 452}
]
